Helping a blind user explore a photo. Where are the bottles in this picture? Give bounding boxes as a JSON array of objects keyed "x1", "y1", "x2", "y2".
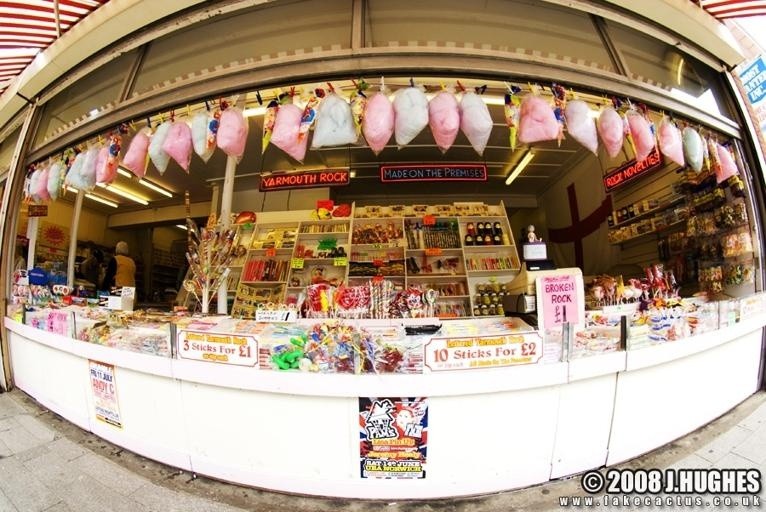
[{"x1": 473, "y1": 276, "x2": 508, "y2": 316}]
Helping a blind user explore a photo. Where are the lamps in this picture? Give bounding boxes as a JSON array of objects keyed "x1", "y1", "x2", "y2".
[
  {"x1": 504, "y1": 146, "x2": 535, "y2": 186},
  {"x1": 62, "y1": 183, "x2": 118, "y2": 211},
  {"x1": 96, "y1": 177, "x2": 149, "y2": 208},
  {"x1": 117, "y1": 166, "x2": 173, "y2": 200},
  {"x1": 176, "y1": 224, "x2": 194, "y2": 234}
]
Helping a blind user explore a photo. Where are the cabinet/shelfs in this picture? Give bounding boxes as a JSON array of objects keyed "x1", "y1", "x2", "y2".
[{"x1": 177, "y1": 200, "x2": 523, "y2": 319}]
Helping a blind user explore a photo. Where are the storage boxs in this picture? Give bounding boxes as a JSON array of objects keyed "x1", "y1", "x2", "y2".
[
  {"x1": 108, "y1": 297, "x2": 121, "y2": 310},
  {"x1": 524, "y1": 242, "x2": 547, "y2": 260}
]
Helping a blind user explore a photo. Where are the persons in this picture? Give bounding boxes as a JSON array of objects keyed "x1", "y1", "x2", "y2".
[
  {"x1": 14, "y1": 243, "x2": 26, "y2": 269},
  {"x1": 99, "y1": 240, "x2": 137, "y2": 292},
  {"x1": 527, "y1": 224, "x2": 542, "y2": 243},
  {"x1": 396, "y1": 409, "x2": 414, "y2": 431},
  {"x1": 272, "y1": 321, "x2": 403, "y2": 374}
]
